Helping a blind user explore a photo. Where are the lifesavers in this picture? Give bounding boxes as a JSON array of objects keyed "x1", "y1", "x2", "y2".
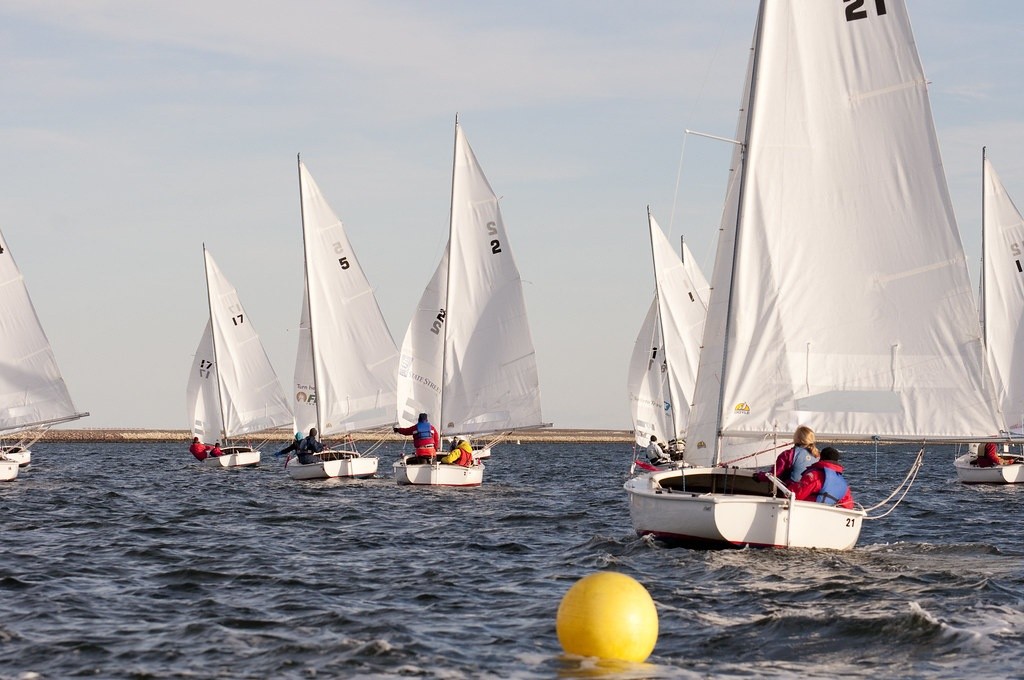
[{"x1": 285, "y1": 455, "x2": 292, "y2": 468}]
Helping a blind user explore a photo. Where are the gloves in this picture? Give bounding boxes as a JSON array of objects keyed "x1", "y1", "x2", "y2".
[
  {"x1": 274, "y1": 451, "x2": 282, "y2": 456},
  {"x1": 393, "y1": 428, "x2": 398, "y2": 433},
  {"x1": 751, "y1": 473, "x2": 760, "y2": 483}
]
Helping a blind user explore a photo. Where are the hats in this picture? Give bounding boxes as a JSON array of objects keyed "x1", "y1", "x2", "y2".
[
  {"x1": 419, "y1": 413, "x2": 427, "y2": 420},
  {"x1": 193, "y1": 437, "x2": 199, "y2": 441},
  {"x1": 658, "y1": 442, "x2": 665, "y2": 449},
  {"x1": 310, "y1": 428, "x2": 317, "y2": 436},
  {"x1": 820, "y1": 446, "x2": 839, "y2": 461},
  {"x1": 651, "y1": 435, "x2": 657, "y2": 441},
  {"x1": 295, "y1": 432, "x2": 303, "y2": 440}
]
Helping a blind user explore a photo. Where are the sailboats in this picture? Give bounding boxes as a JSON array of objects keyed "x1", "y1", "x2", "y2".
[
  {"x1": 391, "y1": 111, "x2": 554, "y2": 485},
  {"x1": 953, "y1": 144, "x2": 1024, "y2": 483},
  {"x1": 186, "y1": 242, "x2": 293, "y2": 466},
  {"x1": 285, "y1": 153, "x2": 400, "y2": 480},
  {"x1": 623, "y1": 0, "x2": 1012, "y2": 554},
  {"x1": 0, "y1": 228, "x2": 90, "y2": 481}
]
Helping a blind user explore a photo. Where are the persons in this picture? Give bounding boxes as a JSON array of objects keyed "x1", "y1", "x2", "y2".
[
  {"x1": 752, "y1": 427, "x2": 820, "y2": 498},
  {"x1": 297, "y1": 428, "x2": 327, "y2": 465},
  {"x1": 448, "y1": 437, "x2": 459, "y2": 452},
  {"x1": 274, "y1": 432, "x2": 305, "y2": 456},
  {"x1": 788, "y1": 447, "x2": 854, "y2": 510},
  {"x1": 646, "y1": 435, "x2": 686, "y2": 466},
  {"x1": 977, "y1": 442, "x2": 1014, "y2": 468},
  {"x1": 209, "y1": 443, "x2": 226, "y2": 457},
  {"x1": 189, "y1": 437, "x2": 216, "y2": 462},
  {"x1": 441, "y1": 440, "x2": 472, "y2": 466},
  {"x1": 393, "y1": 413, "x2": 439, "y2": 465}
]
